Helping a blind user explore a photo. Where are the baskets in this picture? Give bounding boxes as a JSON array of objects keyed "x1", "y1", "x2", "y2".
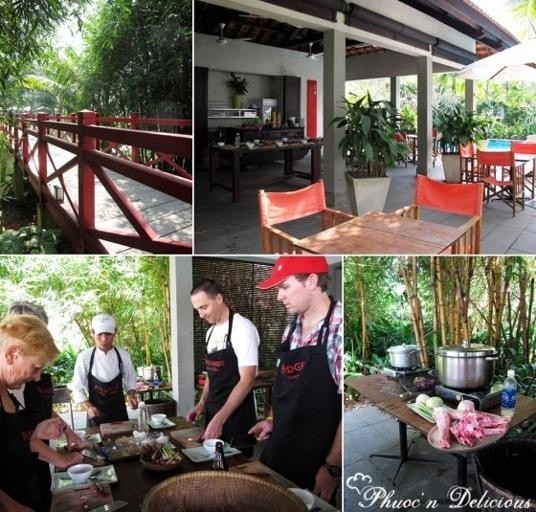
[{"x1": 140, "y1": 470, "x2": 306, "y2": 512}]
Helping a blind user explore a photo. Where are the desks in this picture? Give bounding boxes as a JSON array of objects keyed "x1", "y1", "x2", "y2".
[
  {"x1": 344, "y1": 361, "x2": 535, "y2": 509},
  {"x1": 193, "y1": 371, "x2": 285, "y2": 422},
  {"x1": 42, "y1": 371, "x2": 179, "y2": 432},
  {"x1": 210, "y1": 133, "x2": 328, "y2": 204}
]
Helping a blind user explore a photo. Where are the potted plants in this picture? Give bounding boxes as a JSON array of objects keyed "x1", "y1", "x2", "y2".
[
  {"x1": 325, "y1": 88, "x2": 413, "y2": 217},
  {"x1": 429, "y1": 96, "x2": 496, "y2": 183},
  {"x1": 223, "y1": 74, "x2": 251, "y2": 111}
]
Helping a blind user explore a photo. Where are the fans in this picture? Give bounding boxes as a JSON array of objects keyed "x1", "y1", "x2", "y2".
[
  {"x1": 199, "y1": 21, "x2": 253, "y2": 52},
  {"x1": 288, "y1": 40, "x2": 331, "y2": 63}
]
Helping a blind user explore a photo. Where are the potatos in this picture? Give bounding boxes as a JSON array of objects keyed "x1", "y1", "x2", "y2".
[{"x1": 416, "y1": 393, "x2": 443, "y2": 409}]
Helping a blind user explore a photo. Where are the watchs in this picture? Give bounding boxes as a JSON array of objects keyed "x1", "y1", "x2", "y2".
[{"x1": 321, "y1": 460, "x2": 342, "y2": 479}]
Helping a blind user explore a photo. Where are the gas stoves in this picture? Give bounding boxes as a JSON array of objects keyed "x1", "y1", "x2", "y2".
[
  {"x1": 382, "y1": 366, "x2": 430, "y2": 380},
  {"x1": 434, "y1": 384, "x2": 504, "y2": 410}
]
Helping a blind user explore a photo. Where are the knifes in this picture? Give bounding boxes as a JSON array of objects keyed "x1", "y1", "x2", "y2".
[
  {"x1": 90, "y1": 499, "x2": 128, "y2": 511},
  {"x1": 63, "y1": 444, "x2": 97, "y2": 459},
  {"x1": 229, "y1": 430, "x2": 272, "y2": 449}
]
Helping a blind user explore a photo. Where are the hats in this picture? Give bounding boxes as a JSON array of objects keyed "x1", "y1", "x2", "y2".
[
  {"x1": 255, "y1": 256, "x2": 329, "y2": 290},
  {"x1": 92, "y1": 313, "x2": 116, "y2": 336}
]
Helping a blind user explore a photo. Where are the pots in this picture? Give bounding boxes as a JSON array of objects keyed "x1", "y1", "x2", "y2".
[
  {"x1": 143, "y1": 364, "x2": 161, "y2": 381},
  {"x1": 385, "y1": 342, "x2": 420, "y2": 368},
  {"x1": 433, "y1": 340, "x2": 500, "y2": 390}
]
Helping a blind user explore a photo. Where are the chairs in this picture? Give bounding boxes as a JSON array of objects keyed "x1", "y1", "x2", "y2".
[
  {"x1": 388, "y1": 124, "x2": 536, "y2": 253},
  {"x1": 255, "y1": 176, "x2": 358, "y2": 254}
]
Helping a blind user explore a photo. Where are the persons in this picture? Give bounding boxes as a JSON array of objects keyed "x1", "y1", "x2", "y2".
[
  {"x1": 7, "y1": 299, "x2": 86, "y2": 468},
  {"x1": 70, "y1": 313, "x2": 138, "y2": 428},
  {"x1": 246, "y1": 256, "x2": 343, "y2": 507},
  {"x1": 0, "y1": 312, "x2": 64, "y2": 512},
  {"x1": 184, "y1": 278, "x2": 261, "y2": 458}
]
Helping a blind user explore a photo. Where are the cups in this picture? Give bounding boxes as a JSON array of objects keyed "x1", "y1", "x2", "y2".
[
  {"x1": 151, "y1": 414, "x2": 166, "y2": 424},
  {"x1": 198, "y1": 374, "x2": 206, "y2": 385},
  {"x1": 204, "y1": 438, "x2": 224, "y2": 454},
  {"x1": 143, "y1": 385, "x2": 149, "y2": 389},
  {"x1": 287, "y1": 487, "x2": 315, "y2": 509}
]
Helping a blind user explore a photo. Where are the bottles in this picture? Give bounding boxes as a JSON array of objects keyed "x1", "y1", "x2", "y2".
[
  {"x1": 271, "y1": 112, "x2": 282, "y2": 128},
  {"x1": 501, "y1": 370, "x2": 517, "y2": 417},
  {"x1": 153, "y1": 372, "x2": 158, "y2": 388},
  {"x1": 137, "y1": 402, "x2": 148, "y2": 433},
  {"x1": 235, "y1": 133, "x2": 240, "y2": 147},
  {"x1": 212, "y1": 442, "x2": 228, "y2": 471}
]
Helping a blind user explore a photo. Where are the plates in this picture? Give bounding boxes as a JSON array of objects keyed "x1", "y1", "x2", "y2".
[
  {"x1": 406, "y1": 401, "x2": 438, "y2": 423},
  {"x1": 182, "y1": 444, "x2": 242, "y2": 463},
  {"x1": 50, "y1": 463, "x2": 118, "y2": 491},
  {"x1": 426, "y1": 412, "x2": 508, "y2": 452},
  {"x1": 147, "y1": 417, "x2": 177, "y2": 430}
]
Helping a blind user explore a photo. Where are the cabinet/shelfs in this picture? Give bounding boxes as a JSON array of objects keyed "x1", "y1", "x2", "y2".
[{"x1": 205, "y1": 104, "x2": 259, "y2": 119}]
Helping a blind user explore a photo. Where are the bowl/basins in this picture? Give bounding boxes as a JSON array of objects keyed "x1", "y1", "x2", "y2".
[
  {"x1": 301, "y1": 141, "x2": 307, "y2": 144},
  {"x1": 217, "y1": 143, "x2": 224, "y2": 146},
  {"x1": 138, "y1": 451, "x2": 184, "y2": 472},
  {"x1": 282, "y1": 138, "x2": 288, "y2": 142},
  {"x1": 67, "y1": 464, "x2": 92, "y2": 481},
  {"x1": 254, "y1": 140, "x2": 260, "y2": 144}
]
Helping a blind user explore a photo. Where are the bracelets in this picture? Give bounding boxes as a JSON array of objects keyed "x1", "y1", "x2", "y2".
[
  {"x1": 264, "y1": 416, "x2": 274, "y2": 422},
  {"x1": 61, "y1": 421, "x2": 67, "y2": 432}
]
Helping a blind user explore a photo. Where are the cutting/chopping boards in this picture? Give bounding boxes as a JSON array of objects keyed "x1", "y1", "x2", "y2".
[
  {"x1": 99, "y1": 419, "x2": 139, "y2": 437},
  {"x1": 169, "y1": 427, "x2": 206, "y2": 448},
  {"x1": 53, "y1": 483, "x2": 114, "y2": 511},
  {"x1": 54, "y1": 440, "x2": 104, "y2": 471},
  {"x1": 230, "y1": 458, "x2": 337, "y2": 511}
]
